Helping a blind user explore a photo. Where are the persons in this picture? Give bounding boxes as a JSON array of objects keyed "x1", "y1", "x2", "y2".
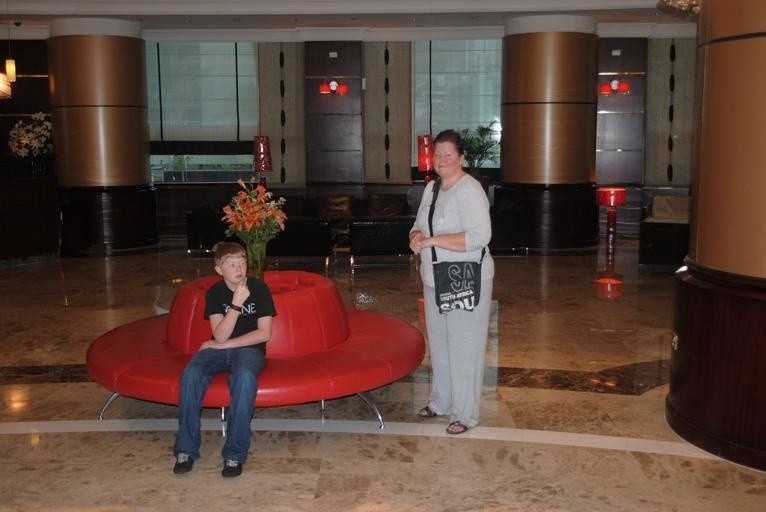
[
  {"x1": 408, "y1": 130, "x2": 495, "y2": 434},
  {"x1": 171, "y1": 241, "x2": 277, "y2": 477}
]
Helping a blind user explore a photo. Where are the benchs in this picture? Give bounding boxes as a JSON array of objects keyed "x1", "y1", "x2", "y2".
[
  {"x1": 79, "y1": 302, "x2": 435, "y2": 438},
  {"x1": 264, "y1": 215, "x2": 341, "y2": 269},
  {"x1": 344, "y1": 215, "x2": 422, "y2": 275}
]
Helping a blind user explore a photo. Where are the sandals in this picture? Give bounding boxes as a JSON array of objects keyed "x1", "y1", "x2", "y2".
[
  {"x1": 446, "y1": 415, "x2": 480, "y2": 435},
  {"x1": 416, "y1": 402, "x2": 442, "y2": 419}
]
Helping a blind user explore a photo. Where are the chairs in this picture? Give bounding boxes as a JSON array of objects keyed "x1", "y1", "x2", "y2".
[
  {"x1": 182, "y1": 206, "x2": 233, "y2": 258},
  {"x1": 486, "y1": 205, "x2": 532, "y2": 259}
]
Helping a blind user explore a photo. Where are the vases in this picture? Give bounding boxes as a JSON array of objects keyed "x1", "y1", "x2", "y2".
[
  {"x1": 25, "y1": 153, "x2": 48, "y2": 177},
  {"x1": 245, "y1": 238, "x2": 268, "y2": 279}
]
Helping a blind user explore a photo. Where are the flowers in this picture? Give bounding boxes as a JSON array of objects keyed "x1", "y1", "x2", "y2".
[
  {"x1": 216, "y1": 176, "x2": 293, "y2": 272},
  {"x1": 3, "y1": 109, "x2": 58, "y2": 160}
]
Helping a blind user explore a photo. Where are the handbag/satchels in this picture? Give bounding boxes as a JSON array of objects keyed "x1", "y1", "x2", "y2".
[{"x1": 430, "y1": 258, "x2": 484, "y2": 317}]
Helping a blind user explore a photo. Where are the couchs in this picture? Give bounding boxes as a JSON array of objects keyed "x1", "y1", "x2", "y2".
[{"x1": 263, "y1": 192, "x2": 411, "y2": 250}]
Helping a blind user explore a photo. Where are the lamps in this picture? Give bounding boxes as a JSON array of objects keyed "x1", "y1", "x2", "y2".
[
  {"x1": 598, "y1": 76, "x2": 631, "y2": 97},
  {"x1": 416, "y1": 132, "x2": 435, "y2": 186},
  {"x1": 593, "y1": 236, "x2": 626, "y2": 300},
  {"x1": 250, "y1": 136, "x2": 275, "y2": 190},
  {"x1": 3, "y1": 0, "x2": 20, "y2": 85},
  {"x1": 596, "y1": 186, "x2": 626, "y2": 236},
  {"x1": 318, "y1": 76, "x2": 347, "y2": 97}
]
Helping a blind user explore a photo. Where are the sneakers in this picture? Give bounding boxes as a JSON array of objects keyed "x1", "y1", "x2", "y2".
[
  {"x1": 172, "y1": 444, "x2": 196, "y2": 475},
  {"x1": 220, "y1": 448, "x2": 247, "y2": 477}
]
